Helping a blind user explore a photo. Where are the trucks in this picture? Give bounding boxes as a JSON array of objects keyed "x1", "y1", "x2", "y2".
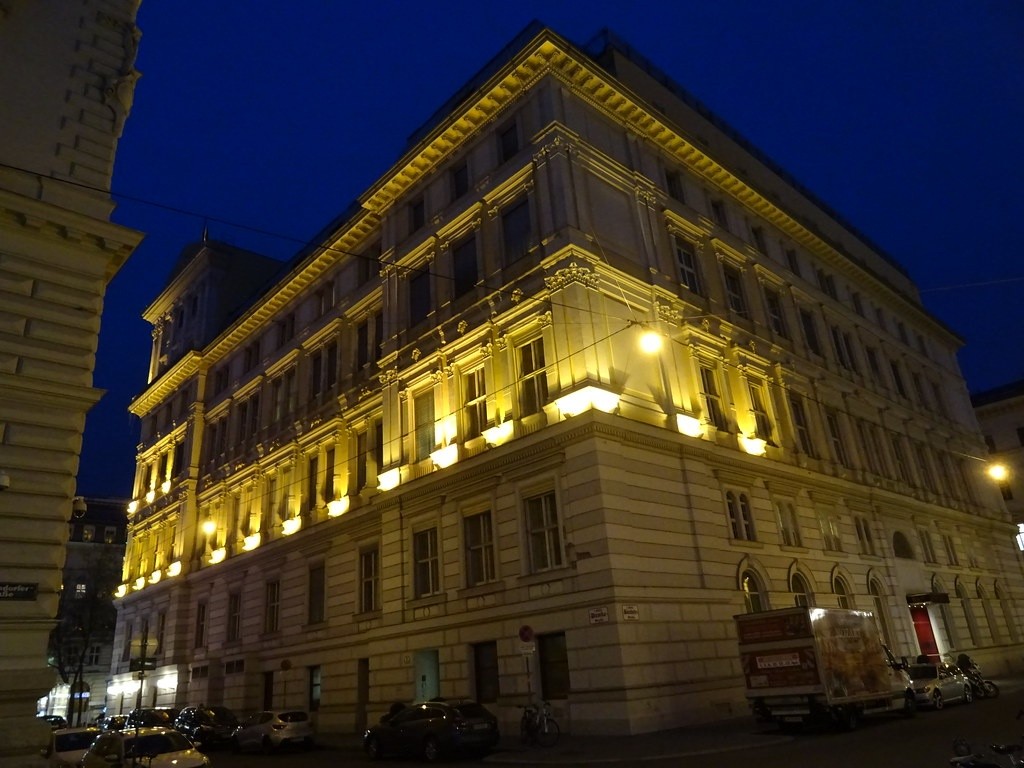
[{"x1": 733, "y1": 606, "x2": 918, "y2": 730}]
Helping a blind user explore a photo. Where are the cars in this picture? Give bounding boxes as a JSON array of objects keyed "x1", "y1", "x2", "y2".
[
  {"x1": 365, "y1": 701, "x2": 500, "y2": 761},
  {"x1": 43, "y1": 705, "x2": 238, "y2": 764},
  {"x1": 81, "y1": 727, "x2": 211, "y2": 768},
  {"x1": 904, "y1": 662, "x2": 973, "y2": 709},
  {"x1": 231, "y1": 709, "x2": 313, "y2": 755}
]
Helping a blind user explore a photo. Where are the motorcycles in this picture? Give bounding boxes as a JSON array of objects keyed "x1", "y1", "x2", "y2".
[{"x1": 963, "y1": 664, "x2": 1000, "y2": 700}]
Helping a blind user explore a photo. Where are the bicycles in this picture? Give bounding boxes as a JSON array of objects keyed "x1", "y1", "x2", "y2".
[{"x1": 521, "y1": 702, "x2": 560, "y2": 748}]
